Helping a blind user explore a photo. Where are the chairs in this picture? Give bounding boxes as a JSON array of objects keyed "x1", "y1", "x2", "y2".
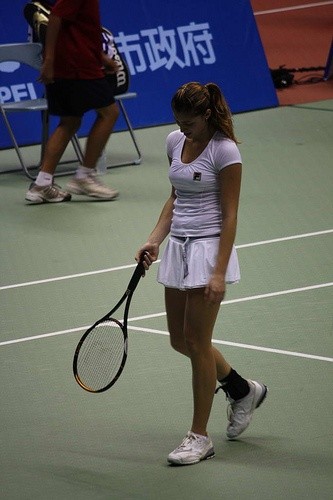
[
  {"x1": 74, "y1": 93, "x2": 143, "y2": 168},
  {"x1": 0, "y1": 44, "x2": 82, "y2": 180}
]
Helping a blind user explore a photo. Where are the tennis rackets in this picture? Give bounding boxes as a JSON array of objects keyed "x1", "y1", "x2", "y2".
[{"x1": 73, "y1": 250, "x2": 151, "y2": 394}]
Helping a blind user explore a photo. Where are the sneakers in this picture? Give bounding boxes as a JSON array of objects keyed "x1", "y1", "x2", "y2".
[
  {"x1": 65, "y1": 172, "x2": 119, "y2": 200},
  {"x1": 226, "y1": 379, "x2": 268, "y2": 439},
  {"x1": 166, "y1": 430, "x2": 215, "y2": 465},
  {"x1": 25, "y1": 181, "x2": 72, "y2": 203}
]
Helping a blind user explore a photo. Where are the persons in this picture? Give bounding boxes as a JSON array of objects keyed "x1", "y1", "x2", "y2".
[
  {"x1": 135, "y1": 82, "x2": 269, "y2": 465},
  {"x1": 23, "y1": 0, "x2": 120, "y2": 202}
]
